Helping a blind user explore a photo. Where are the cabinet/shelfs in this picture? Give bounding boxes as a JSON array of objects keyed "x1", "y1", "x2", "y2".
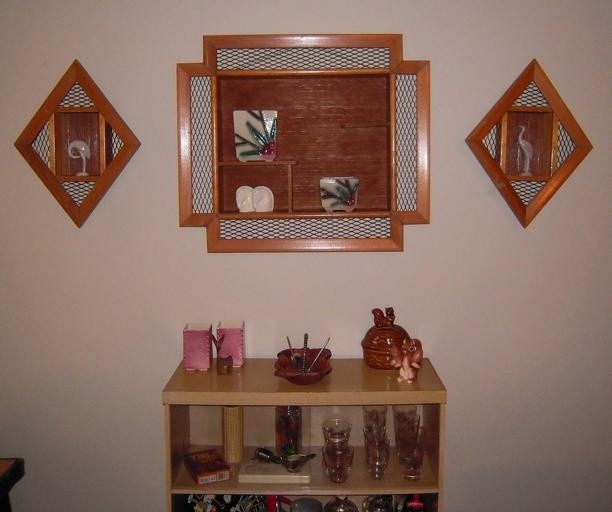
[{"x1": 162, "y1": 357, "x2": 447, "y2": 512}]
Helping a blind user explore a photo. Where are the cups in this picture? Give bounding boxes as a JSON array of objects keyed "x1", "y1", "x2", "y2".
[
  {"x1": 223, "y1": 403, "x2": 245, "y2": 465},
  {"x1": 321, "y1": 404, "x2": 427, "y2": 482}
]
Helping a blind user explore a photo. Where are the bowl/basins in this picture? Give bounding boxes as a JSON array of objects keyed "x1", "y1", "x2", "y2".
[{"x1": 275, "y1": 346, "x2": 332, "y2": 386}]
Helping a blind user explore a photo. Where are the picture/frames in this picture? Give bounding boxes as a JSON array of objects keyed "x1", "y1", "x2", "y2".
[
  {"x1": 177, "y1": 33, "x2": 430, "y2": 254},
  {"x1": 465, "y1": 59, "x2": 594, "y2": 229},
  {"x1": 14, "y1": 59, "x2": 142, "y2": 229}
]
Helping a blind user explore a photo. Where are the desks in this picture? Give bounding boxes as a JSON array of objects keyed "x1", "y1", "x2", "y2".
[{"x1": 0, "y1": 458, "x2": 25, "y2": 512}]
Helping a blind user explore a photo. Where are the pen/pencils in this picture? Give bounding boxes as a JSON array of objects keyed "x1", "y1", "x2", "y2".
[
  {"x1": 308, "y1": 336, "x2": 330, "y2": 373},
  {"x1": 286, "y1": 334, "x2": 299, "y2": 373},
  {"x1": 302, "y1": 333, "x2": 308, "y2": 375}
]
